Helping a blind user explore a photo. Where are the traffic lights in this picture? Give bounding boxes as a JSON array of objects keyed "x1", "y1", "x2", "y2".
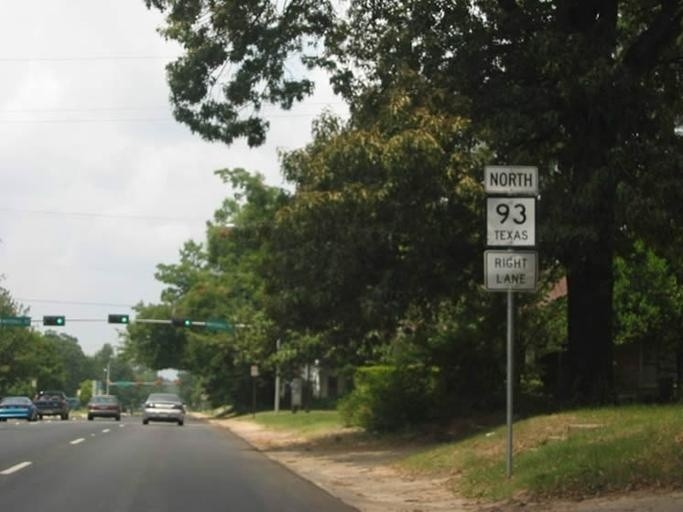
[
  {"x1": 107, "y1": 314, "x2": 127, "y2": 323},
  {"x1": 171, "y1": 317, "x2": 192, "y2": 328},
  {"x1": 41, "y1": 316, "x2": 64, "y2": 326}
]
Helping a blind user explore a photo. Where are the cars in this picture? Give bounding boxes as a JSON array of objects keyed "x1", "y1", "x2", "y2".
[
  {"x1": 141, "y1": 393, "x2": 185, "y2": 426},
  {"x1": 87, "y1": 395, "x2": 121, "y2": 421},
  {"x1": 0, "y1": 396, "x2": 37, "y2": 422}
]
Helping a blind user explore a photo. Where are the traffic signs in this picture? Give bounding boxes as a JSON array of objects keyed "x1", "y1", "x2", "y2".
[{"x1": 0, "y1": 317, "x2": 30, "y2": 327}]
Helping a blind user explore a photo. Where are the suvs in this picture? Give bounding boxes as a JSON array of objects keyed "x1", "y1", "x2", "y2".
[{"x1": 33, "y1": 390, "x2": 70, "y2": 421}]
